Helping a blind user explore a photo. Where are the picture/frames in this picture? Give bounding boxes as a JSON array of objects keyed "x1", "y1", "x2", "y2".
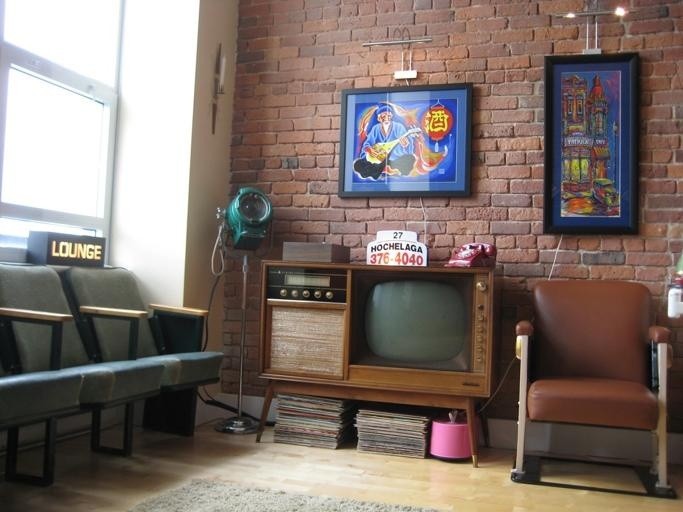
[
  {"x1": 338, "y1": 81, "x2": 472, "y2": 200},
  {"x1": 541, "y1": 50, "x2": 641, "y2": 235}
]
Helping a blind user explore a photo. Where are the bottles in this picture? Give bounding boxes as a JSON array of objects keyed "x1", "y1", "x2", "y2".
[{"x1": 665, "y1": 269, "x2": 683, "y2": 319}]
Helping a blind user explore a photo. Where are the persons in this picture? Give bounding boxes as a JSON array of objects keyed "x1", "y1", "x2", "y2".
[{"x1": 353, "y1": 106, "x2": 418, "y2": 181}]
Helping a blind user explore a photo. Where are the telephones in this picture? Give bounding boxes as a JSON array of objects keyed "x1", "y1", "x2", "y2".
[{"x1": 444, "y1": 243, "x2": 497, "y2": 268}]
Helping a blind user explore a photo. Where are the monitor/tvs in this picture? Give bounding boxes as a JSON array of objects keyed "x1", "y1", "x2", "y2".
[{"x1": 343, "y1": 263, "x2": 495, "y2": 398}]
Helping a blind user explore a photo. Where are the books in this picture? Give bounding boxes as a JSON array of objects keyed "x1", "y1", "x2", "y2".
[{"x1": 272, "y1": 393, "x2": 430, "y2": 459}]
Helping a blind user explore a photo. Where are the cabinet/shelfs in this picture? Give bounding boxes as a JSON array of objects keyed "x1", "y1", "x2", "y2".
[{"x1": 252, "y1": 258, "x2": 495, "y2": 468}]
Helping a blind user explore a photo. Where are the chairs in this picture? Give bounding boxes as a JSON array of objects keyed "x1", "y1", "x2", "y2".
[
  {"x1": 511, "y1": 279, "x2": 673, "y2": 498},
  {"x1": 1, "y1": 264, "x2": 223, "y2": 488}
]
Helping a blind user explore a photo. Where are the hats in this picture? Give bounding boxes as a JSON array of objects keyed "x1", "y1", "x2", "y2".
[{"x1": 376, "y1": 104, "x2": 391, "y2": 114}]
